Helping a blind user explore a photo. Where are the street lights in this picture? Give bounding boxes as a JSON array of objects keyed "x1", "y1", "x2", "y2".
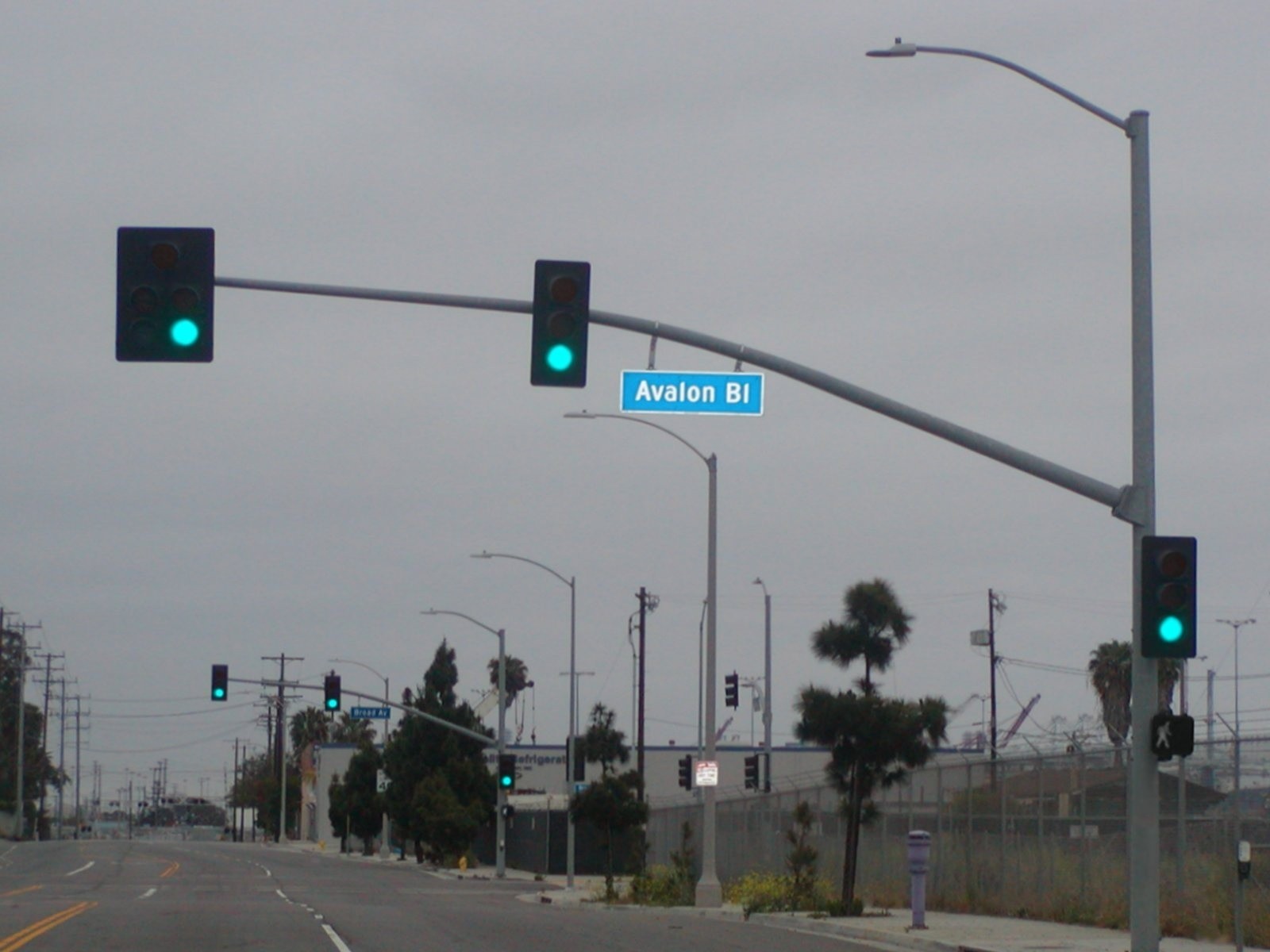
[
  {"x1": 328, "y1": 657, "x2": 391, "y2": 858},
  {"x1": 420, "y1": 606, "x2": 508, "y2": 877},
  {"x1": 1217, "y1": 617, "x2": 1257, "y2": 791},
  {"x1": 469, "y1": 551, "x2": 582, "y2": 910},
  {"x1": 864, "y1": 37, "x2": 1162, "y2": 952},
  {"x1": 564, "y1": 410, "x2": 724, "y2": 909},
  {"x1": 752, "y1": 575, "x2": 772, "y2": 794}
]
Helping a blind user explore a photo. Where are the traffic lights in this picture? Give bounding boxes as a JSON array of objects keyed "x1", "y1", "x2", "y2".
[
  {"x1": 210, "y1": 663, "x2": 229, "y2": 701},
  {"x1": 530, "y1": 259, "x2": 592, "y2": 389},
  {"x1": 499, "y1": 752, "x2": 518, "y2": 794},
  {"x1": 115, "y1": 226, "x2": 215, "y2": 364},
  {"x1": 1142, "y1": 534, "x2": 1196, "y2": 662},
  {"x1": 324, "y1": 676, "x2": 328, "y2": 715},
  {"x1": 745, "y1": 754, "x2": 759, "y2": 790},
  {"x1": 678, "y1": 754, "x2": 692, "y2": 790},
  {"x1": 724, "y1": 670, "x2": 738, "y2": 707}
]
